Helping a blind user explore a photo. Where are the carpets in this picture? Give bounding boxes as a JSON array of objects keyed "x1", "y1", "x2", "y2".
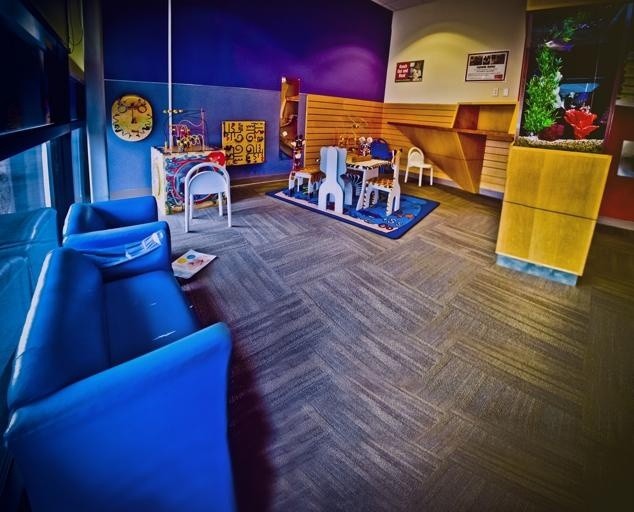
[{"x1": 265, "y1": 181, "x2": 440, "y2": 239}]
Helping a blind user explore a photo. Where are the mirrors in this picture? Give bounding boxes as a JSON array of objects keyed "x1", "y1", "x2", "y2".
[{"x1": 279, "y1": 76, "x2": 300, "y2": 161}]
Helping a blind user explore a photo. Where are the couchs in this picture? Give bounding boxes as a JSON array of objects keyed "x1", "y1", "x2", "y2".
[{"x1": 3, "y1": 246, "x2": 238, "y2": 512}]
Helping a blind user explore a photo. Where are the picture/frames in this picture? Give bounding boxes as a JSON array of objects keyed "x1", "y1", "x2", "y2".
[
  {"x1": 395, "y1": 60, "x2": 424, "y2": 83},
  {"x1": 465, "y1": 50, "x2": 508, "y2": 81}
]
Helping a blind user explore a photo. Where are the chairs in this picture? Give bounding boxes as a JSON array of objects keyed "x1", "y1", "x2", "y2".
[
  {"x1": 62, "y1": 194, "x2": 172, "y2": 283},
  {"x1": 317, "y1": 137, "x2": 434, "y2": 215},
  {"x1": 184, "y1": 161, "x2": 231, "y2": 233}
]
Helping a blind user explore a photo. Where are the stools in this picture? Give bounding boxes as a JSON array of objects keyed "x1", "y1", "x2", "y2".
[{"x1": 288, "y1": 164, "x2": 324, "y2": 200}]
